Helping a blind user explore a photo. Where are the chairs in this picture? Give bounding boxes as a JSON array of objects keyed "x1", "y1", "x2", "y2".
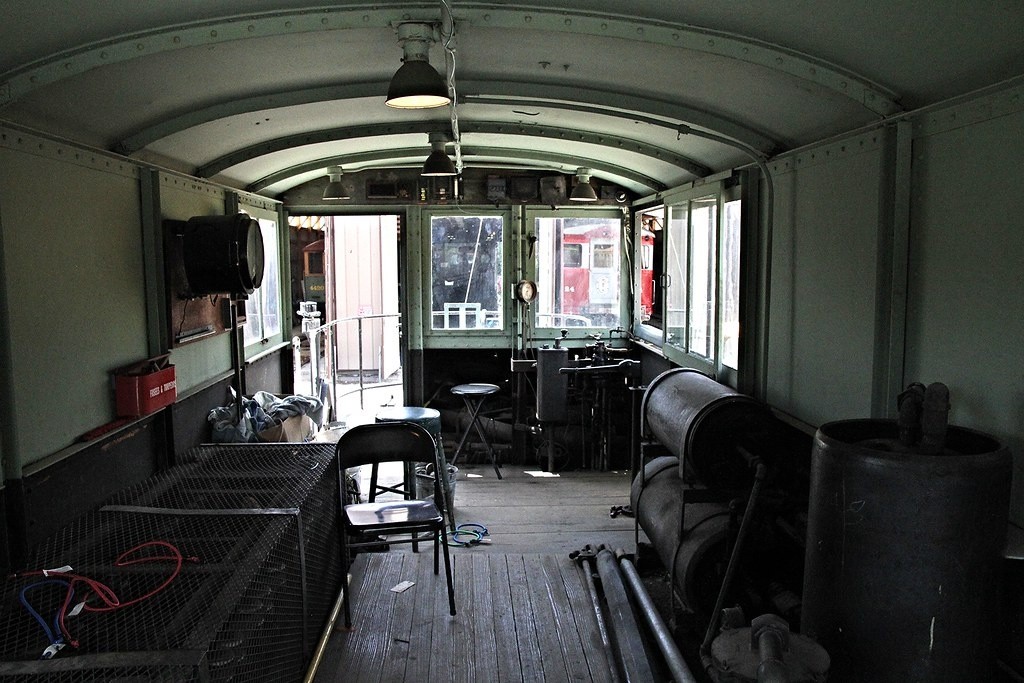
[{"x1": 336, "y1": 421, "x2": 457, "y2": 631}]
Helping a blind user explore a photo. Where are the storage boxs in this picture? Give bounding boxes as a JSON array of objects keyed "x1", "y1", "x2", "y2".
[
  {"x1": 0, "y1": 441, "x2": 350, "y2": 683},
  {"x1": 213, "y1": 392, "x2": 325, "y2": 441}
]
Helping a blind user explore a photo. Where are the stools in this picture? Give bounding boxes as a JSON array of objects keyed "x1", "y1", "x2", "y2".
[{"x1": 446, "y1": 383, "x2": 502, "y2": 482}]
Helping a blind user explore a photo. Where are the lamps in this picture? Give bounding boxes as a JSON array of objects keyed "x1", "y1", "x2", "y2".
[
  {"x1": 419, "y1": 131, "x2": 458, "y2": 177},
  {"x1": 382, "y1": 17, "x2": 453, "y2": 110},
  {"x1": 568, "y1": 166, "x2": 600, "y2": 203},
  {"x1": 322, "y1": 164, "x2": 350, "y2": 200}
]
